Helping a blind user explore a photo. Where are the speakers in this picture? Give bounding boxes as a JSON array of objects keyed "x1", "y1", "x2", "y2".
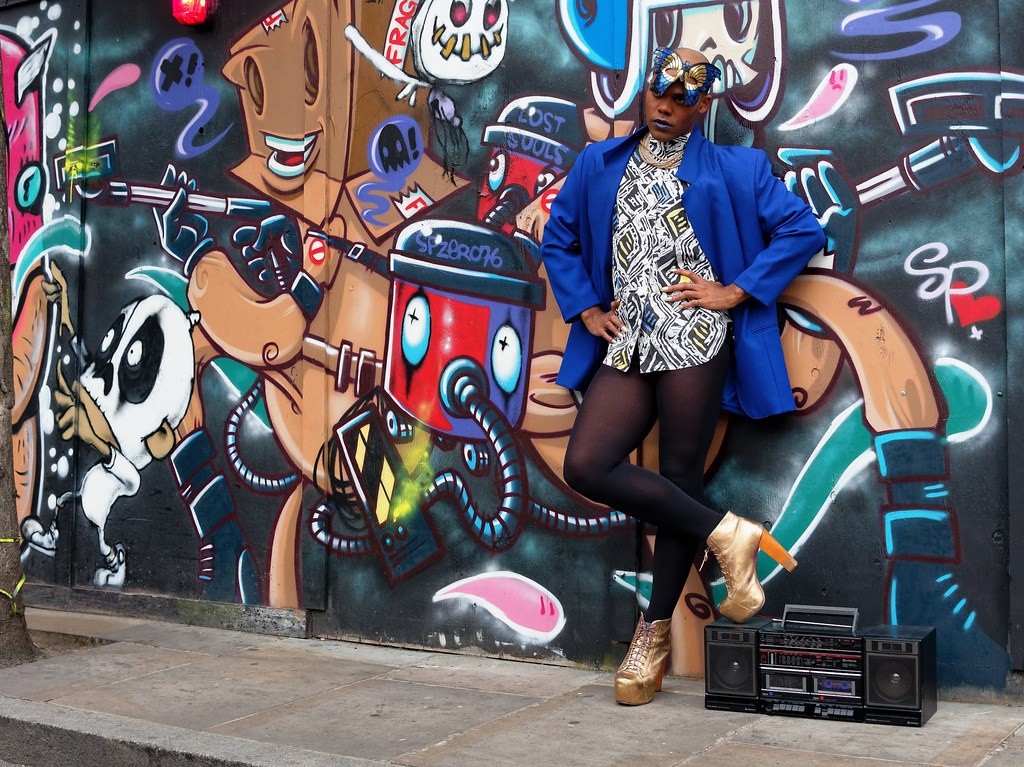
[
  {"x1": 703, "y1": 616, "x2": 773, "y2": 698},
  {"x1": 866, "y1": 623, "x2": 938, "y2": 709}
]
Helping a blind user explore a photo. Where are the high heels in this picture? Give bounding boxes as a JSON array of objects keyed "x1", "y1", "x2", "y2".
[
  {"x1": 699, "y1": 510, "x2": 798, "y2": 623},
  {"x1": 615, "y1": 611, "x2": 673, "y2": 705}
]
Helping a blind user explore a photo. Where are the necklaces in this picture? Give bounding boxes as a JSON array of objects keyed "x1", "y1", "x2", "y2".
[{"x1": 638, "y1": 133, "x2": 683, "y2": 169}]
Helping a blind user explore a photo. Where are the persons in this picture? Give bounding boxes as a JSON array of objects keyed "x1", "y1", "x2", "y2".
[{"x1": 540, "y1": 47, "x2": 827, "y2": 705}]
